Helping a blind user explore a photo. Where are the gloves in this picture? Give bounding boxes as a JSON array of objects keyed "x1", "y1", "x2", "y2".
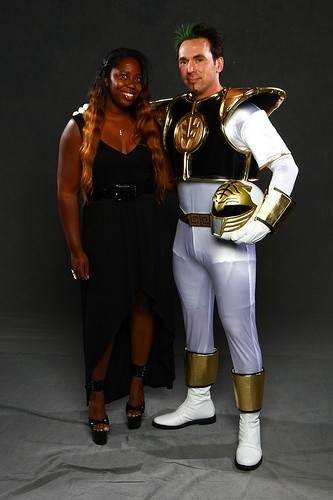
[{"x1": 230, "y1": 188, "x2": 295, "y2": 244}]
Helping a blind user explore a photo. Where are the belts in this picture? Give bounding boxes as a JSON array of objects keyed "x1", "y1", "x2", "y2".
[{"x1": 178, "y1": 205, "x2": 210, "y2": 227}]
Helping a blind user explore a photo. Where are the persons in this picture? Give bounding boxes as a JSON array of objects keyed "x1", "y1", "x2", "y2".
[
  {"x1": 152, "y1": 24, "x2": 299, "y2": 471},
  {"x1": 58, "y1": 48, "x2": 179, "y2": 445}
]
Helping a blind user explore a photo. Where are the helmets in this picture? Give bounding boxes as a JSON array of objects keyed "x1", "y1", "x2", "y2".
[{"x1": 209, "y1": 180, "x2": 265, "y2": 239}]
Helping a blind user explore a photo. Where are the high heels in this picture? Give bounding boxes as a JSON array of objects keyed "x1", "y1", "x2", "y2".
[
  {"x1": 125, "y1": 363, "x2": 147, "y2": 430},
  {"x1": 87, "y1": 379, "x2": 110, "y2": 445}
]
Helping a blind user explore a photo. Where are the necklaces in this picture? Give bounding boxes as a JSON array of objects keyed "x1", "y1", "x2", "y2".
[{"x1": 120, "y1": 129, "x2": 122, "y2": 135}]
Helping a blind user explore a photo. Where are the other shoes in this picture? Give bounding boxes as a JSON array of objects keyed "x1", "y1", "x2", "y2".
[
  {"x1": 152, "y1": 386, "x2": 216, "y2": 429},
  {"x1": 234, "y1": 412, "x2": 263, "y2": 471}
]
